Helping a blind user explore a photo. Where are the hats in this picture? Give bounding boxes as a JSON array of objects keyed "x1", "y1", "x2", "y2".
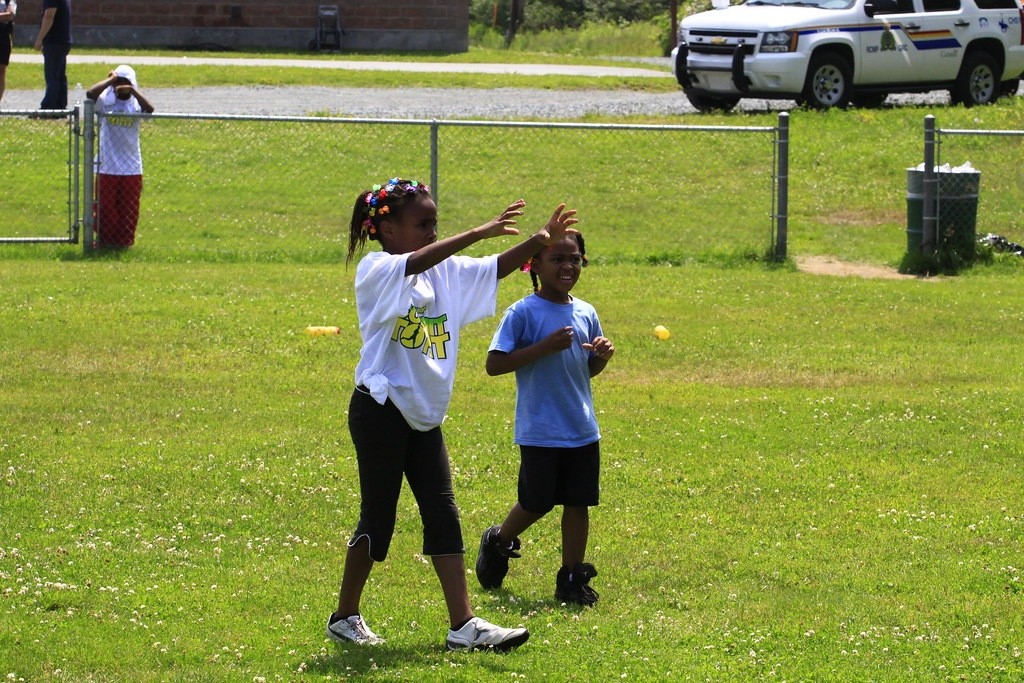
[{"x1": 113, "y1": 64, "x2": 137, "y2": 87}]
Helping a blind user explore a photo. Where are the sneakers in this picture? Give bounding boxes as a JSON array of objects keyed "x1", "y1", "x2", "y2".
[
  {"x1": 444, "y1": 618, "x2": 530, "y2": 653},
  {"x1": 327, "y1": 610, "x2": 387, "y2": 647},
  {"x1": 553, "y1": 562, "x2": 600, "y2": 605},
  {"x1": 476, "y1": 526, "x2": 519, "y2": 589}
]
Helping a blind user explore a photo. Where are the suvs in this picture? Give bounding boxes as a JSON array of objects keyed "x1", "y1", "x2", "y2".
[{"x1": 670, "y1": 0, "x2": 1023, "y2": 116}]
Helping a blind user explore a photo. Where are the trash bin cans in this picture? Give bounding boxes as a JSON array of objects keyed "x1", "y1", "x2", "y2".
[{"x1": 906, "y1": 161, "x2": 981, "y2": 254}]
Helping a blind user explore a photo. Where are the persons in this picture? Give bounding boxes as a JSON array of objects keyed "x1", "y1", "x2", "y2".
[
  {"x1": 474, "y1": 225, "x2": 615, "y2": 610},
  {"x1": 84, "y1": 64, "x2": 157, "y2": 250},
  {"x1": 0, "y1": 0, "x2": 17, "y2": 101},
  {"x1": 29, "y1": 0, "x2": 73, "y2": 121},
  {"x1": 325, "y1": 177, "x2": 584, "y2": 650}
]
